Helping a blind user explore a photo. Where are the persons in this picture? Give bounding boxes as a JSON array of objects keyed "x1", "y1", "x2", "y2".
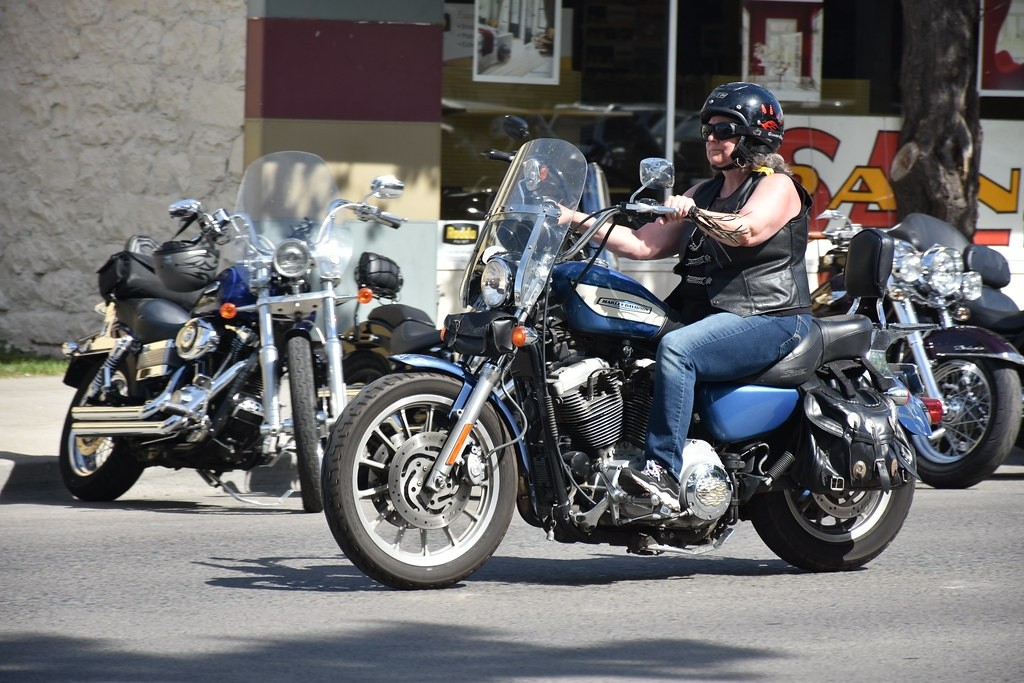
[{"x1": 546, "y1": 82, "x2": 812, "y2": 512}]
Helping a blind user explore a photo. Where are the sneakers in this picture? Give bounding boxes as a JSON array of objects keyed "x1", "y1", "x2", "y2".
[{"x1": 618, "y1": 460, "x2": 682, "y2": 513}]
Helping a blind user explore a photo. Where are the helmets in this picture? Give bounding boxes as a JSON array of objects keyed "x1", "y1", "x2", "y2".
[
  {"x1": 152, "y1": 239, "x2": 220, "y2": 291},
  {"x1": 700, "y1": 81, "x2": 785, "y2": 154}
]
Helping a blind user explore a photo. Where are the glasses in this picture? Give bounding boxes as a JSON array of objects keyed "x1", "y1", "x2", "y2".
[{"x1": 699, "y1": 120, "x2": 743, "y2": 141}]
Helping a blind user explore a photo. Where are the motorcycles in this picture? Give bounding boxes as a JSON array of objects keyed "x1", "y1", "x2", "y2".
[
  {"x1": 321, "y1": 114, "x2": 619, "y2": 455},
  {"x1": 810, "y1": 210, "x2": 1024, "y2": 488},
  {"x1": 60, "y1": 150, "x2": 411, "y2": 512},
  {"x1": 320, "y1": 138, "x2": 947, "y2": 589}
]
